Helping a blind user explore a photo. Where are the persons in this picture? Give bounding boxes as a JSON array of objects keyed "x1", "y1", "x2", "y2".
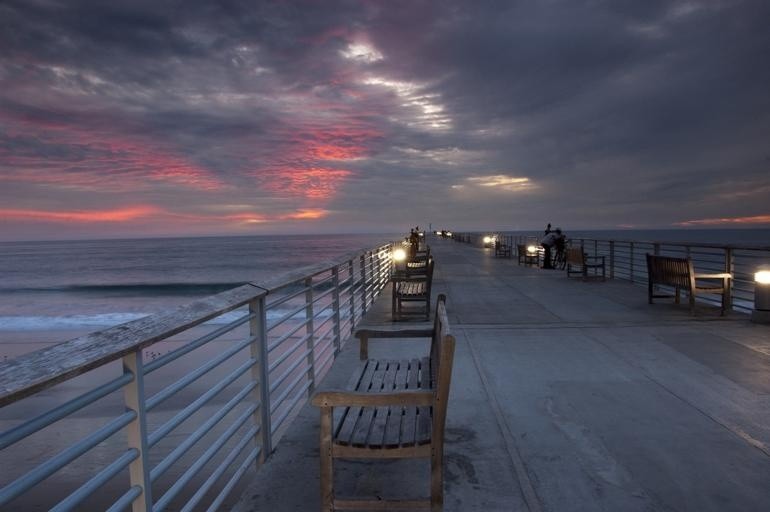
[
  {"x1": 411, "y1": 226, "x2": 420, "y2": 243},
  {"x1": 541, "y1": 229, "x2": 562, "y2": 269},
  {"x1": 545, "y1": 224, "x2": 551, "y2": 235}
]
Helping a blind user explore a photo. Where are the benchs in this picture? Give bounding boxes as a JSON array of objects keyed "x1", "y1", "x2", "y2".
[
  {"x1": 644, "y1": 251, "x2": 733, "y2": 318},
  {"x1": 309, "y1": 244, "x2": 456, "y2": 511},
  {"x1": 492, "y1": 239, "x2": 607, "y2": 281}
]
